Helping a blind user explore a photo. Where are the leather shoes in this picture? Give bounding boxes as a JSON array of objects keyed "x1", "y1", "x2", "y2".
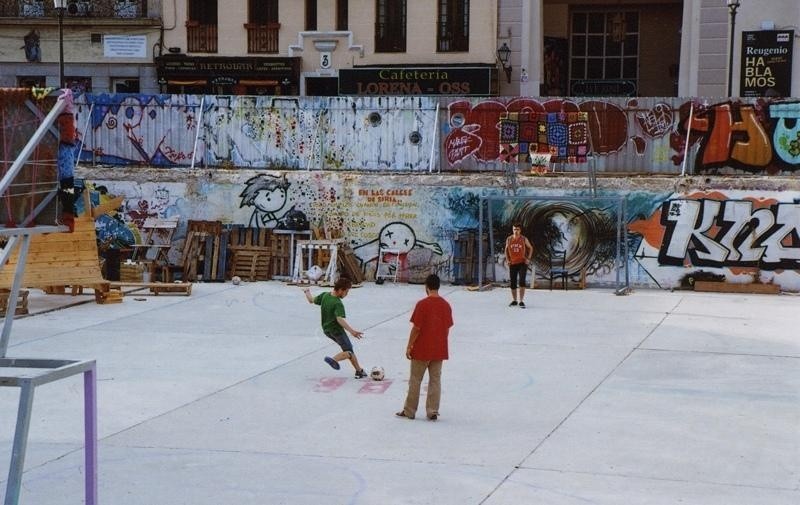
[{"x1": 396, "y1": 410, "x2": 415, "y2": 419}]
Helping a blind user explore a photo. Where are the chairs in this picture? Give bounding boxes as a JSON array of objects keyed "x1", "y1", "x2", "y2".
[{"x1": 549, "y1": 249, "x2": 569, "y2": 291}]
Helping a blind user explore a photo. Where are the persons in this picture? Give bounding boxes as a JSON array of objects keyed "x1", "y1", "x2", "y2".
[
  {"x1": 303, "y1": 277, "x2": 368, "y2": 380},
  {"x1": 503, "y1": 222, "x2": 534, "y2": 309},
  {"x1": 394, "y1": 272, "x2": 454, "y2": 421}
]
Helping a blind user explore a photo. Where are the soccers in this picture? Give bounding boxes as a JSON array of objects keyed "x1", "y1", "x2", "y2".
[
  {"x1": 232, "y1": 276, "x2": 241, "y2": 285},
  {"x1": 371, "y1": 367, "x2": 384, "y2": 381}
]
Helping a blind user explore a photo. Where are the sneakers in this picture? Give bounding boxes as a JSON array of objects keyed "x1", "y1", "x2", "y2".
[
  {"x1": 324, "y1": 356, "x2": 340, "y2": 370},
  {"x1": 355, "y1": 369, "x2": 367, "y2": 379},
  {"x1": 511, "y1": 301, "x2": 518, "y2": 305},
  {"x1": 519, "y1": 302, "x2": 524, "y2": 306}
]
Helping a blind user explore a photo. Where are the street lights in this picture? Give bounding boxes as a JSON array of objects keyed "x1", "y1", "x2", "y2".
[
  {"x1": 726, "y1": 0, "x2": 740, "y2": 99},
  {"x1": 54, "y1": 0, "x2": 68, "y2": 86}
]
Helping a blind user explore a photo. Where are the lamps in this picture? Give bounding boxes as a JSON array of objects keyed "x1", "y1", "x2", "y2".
[{"x1": 498, "y1": 43, "x2": 512, "y2": 84}]
